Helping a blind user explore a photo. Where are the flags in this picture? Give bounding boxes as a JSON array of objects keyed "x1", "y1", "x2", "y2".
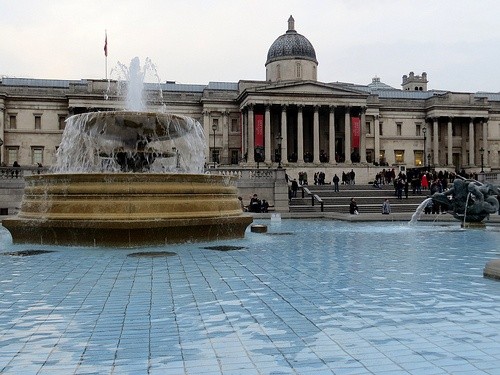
[{"x1": 104, "y1": 34, "x2": 107, "y2": 57}]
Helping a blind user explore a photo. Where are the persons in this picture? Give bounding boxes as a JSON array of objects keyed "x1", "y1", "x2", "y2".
[
  {"x1": 2, "y1": 161, "x2": 22, "y2": 180},
  {"x1": 373, "y1": 168, "x2": 477, "y2": 200},
  {"x1": 379, "y1": 159, "x2": 383, "y2": 166},
  {"x1": 342, "y1": 169, "x2": 355, "y2": 186},
  {"x1": 37, "y1": 162, "x2": 43, "y2": 174},
  {"x1": 424, "y1": 195, "x2": 448, "y2": 215},
  {"x1": 314, "y1": 172, "x2": 325, "y2": 186},
  {"x1": 382, "y1": 198, "x2": 391, "y2": 214},
  {"x1": 333, "y1": 174, "x2": 340, "y2": 192},
  {"x1": 298, "y1": 172, "x2": 307, "y2": 185},
  {"x1": 245, "y1": 145, "x2": 372, "y2": 164},
  {"x1": 349, "y1": 198, "x2": 359, "y2": 214},
  {"x1": 289, "y1": 178, "x2": 298, "y2": 198},
  {"x1": 238, "y1": 194, "x2": 269, "y2": 213}
]
